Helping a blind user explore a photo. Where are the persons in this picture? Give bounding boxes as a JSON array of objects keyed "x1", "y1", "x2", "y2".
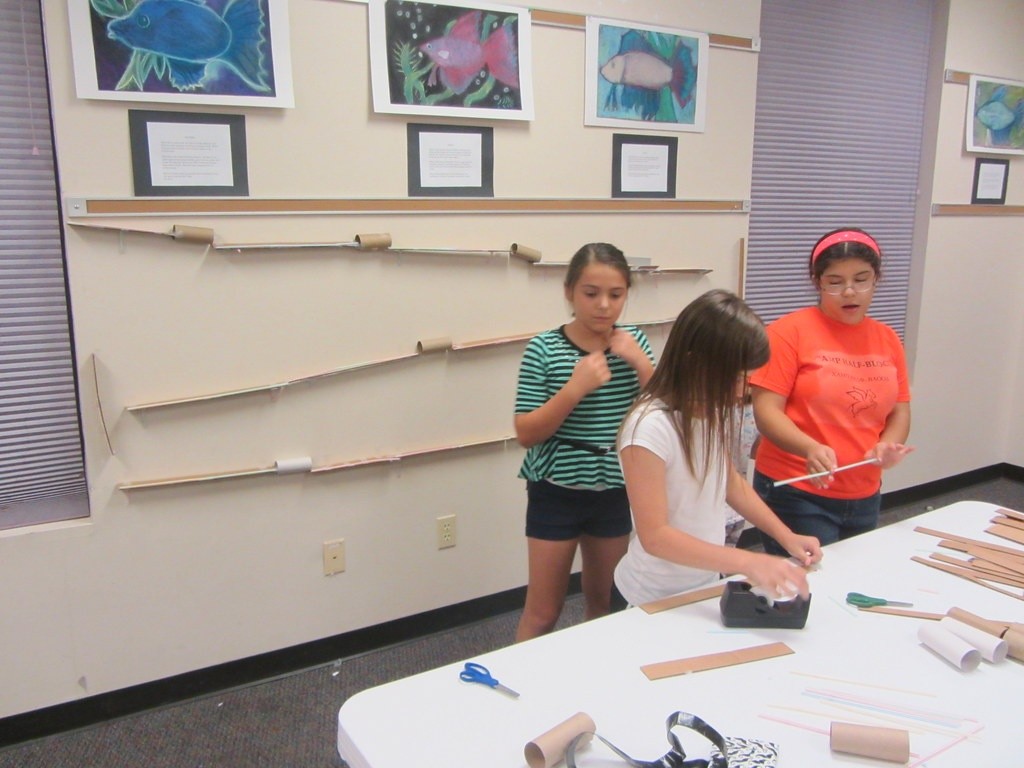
[
  {"x1": 609, "y1": 289, "x2": 823, "y2": 615},
  {"x1": 514, "y1": 243, "x2": 658, "y2": 643},
  {"x1": 748, "y1": 226, "x2": 916, "y2": 558}
]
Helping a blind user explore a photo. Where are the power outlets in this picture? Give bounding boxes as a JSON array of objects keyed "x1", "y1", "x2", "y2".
[{"x1": 436, "y1": 515, "x2": 457, "y2": 550}]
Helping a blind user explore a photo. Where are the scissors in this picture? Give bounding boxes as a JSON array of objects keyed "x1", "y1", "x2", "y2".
[
  {"x1": 845, "y1": 592, "x2": 913, "y2": 608},
  {"x1": 459, "y1": 663, "x2": 520, "y2": 698}
]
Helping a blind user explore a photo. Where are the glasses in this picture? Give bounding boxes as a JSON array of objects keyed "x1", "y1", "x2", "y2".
[
  {"x1": 817, "y1": 273, "x2": 877, "y2": 296},
  {"x1": 737, "y1": 372, "x2": 750, "y2": 385}
]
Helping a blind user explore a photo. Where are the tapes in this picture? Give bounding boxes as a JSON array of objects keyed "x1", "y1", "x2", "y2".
[{"x1": 749, "y1": 586, "x2": 774, "y2": 609}]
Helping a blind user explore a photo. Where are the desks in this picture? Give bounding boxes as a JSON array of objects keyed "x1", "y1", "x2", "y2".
[{"x1": 336, "y1": 501, "x2": 1024, "y2": 768}]
